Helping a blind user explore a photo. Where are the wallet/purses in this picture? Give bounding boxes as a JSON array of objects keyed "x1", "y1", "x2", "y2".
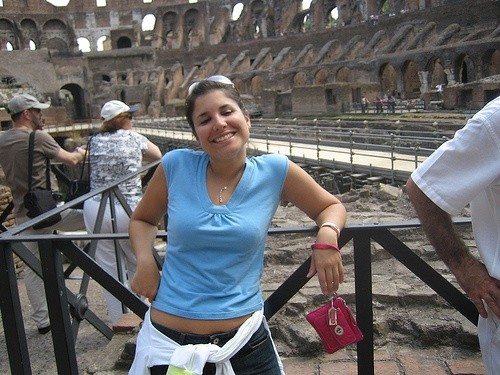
[{"x1": 306, "y1": 244, "x2": 364, "y2": 354}]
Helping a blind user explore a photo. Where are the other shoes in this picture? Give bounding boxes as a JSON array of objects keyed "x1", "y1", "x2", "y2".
[
  {"x1": 39, "y1": 325, "x2": 52, "y2": 334},
  {"x1": 113, "y1": 326, "x2": 134, "y2": 331}
]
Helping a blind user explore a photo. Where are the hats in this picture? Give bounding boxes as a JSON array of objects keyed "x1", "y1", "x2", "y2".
[
  {"x1": 7, "y1": 94, "x2": 50, "y2": 115},
  {"x1": 101, "y1": 99, "x2": 141, "y2": 122}
]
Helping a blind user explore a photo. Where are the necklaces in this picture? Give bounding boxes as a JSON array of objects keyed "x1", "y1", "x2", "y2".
[{"x1": 210, "y1": 157, "x2": 243, "y2": 203}]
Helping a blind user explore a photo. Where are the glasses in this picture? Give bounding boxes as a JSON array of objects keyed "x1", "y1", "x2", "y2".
[
  {"x1": 185, "y1": 74, "x2": 241, "y2": 99},
  {"x1": 126, "y1": 114, "x2": 132, "y2": 120}
]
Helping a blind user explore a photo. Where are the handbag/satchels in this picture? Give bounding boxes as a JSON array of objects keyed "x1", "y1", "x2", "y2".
[
  {"x1": 65, "y1": 133, "x2": 99, "y2": 209},
  {"x1": 23, "y1": 130, "x2": 62, "y2": 230}
]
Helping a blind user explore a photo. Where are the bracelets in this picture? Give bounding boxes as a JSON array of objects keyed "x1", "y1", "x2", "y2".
[{"x1": 319, "y1": 221, "x2": 341, "y2": 240}]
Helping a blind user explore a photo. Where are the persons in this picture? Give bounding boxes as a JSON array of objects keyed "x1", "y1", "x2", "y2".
[
  {"x1": 76, "y1": 99, "x2": 165, "y2": 336},
  {"x1": 123, "y1": 75, "x2": 346, "y2": 375},
  {"x1": 404, "y1": 96, "x2": 500, "y2": 375},
  {"x1": 0, "y1": 92, "x2": 87, "y2": 335},
  {"x1": 360, "y1": 94, "x2": 397, "y2": 113}
]
